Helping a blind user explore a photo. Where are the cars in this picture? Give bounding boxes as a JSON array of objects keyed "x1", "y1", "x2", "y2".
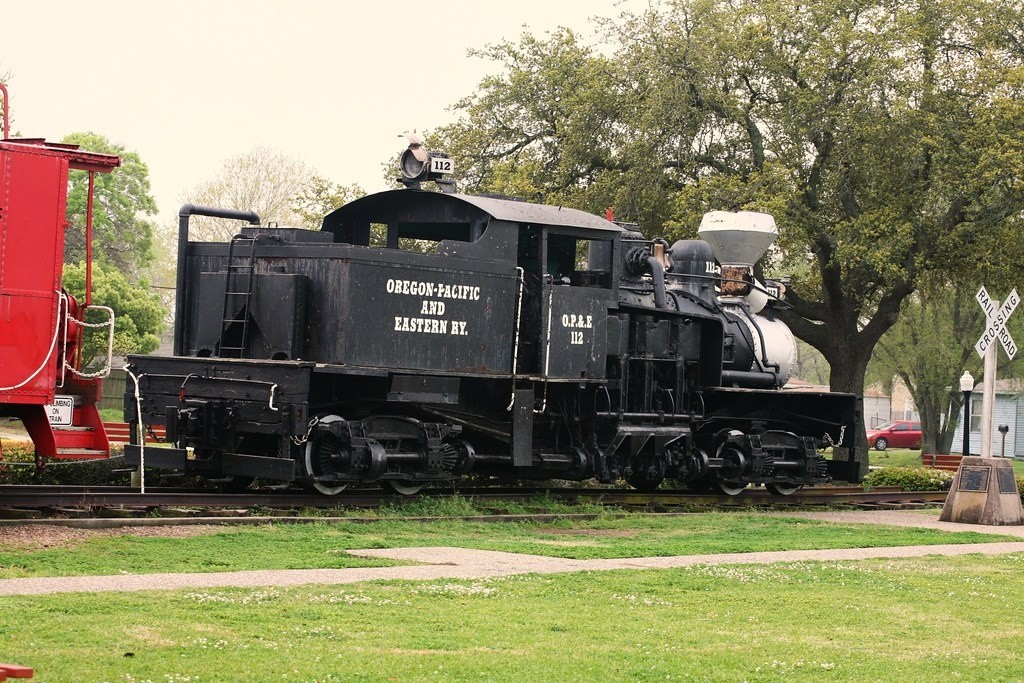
[{"x1": 866, "y1": 420, "x2": 924, "y2": 450}]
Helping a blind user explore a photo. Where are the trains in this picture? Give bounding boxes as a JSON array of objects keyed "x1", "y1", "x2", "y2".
[
  {"x1": 122, "y1": 142, "x2": 868, "y2": 511},
  {"x1": 1, "y1": 83, "x2": 136, "y2": 483}
]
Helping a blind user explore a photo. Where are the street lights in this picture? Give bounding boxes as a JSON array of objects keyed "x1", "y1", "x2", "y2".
[{"x1": 959, "y1": 369, "x2": 975, "y2": 458}]
